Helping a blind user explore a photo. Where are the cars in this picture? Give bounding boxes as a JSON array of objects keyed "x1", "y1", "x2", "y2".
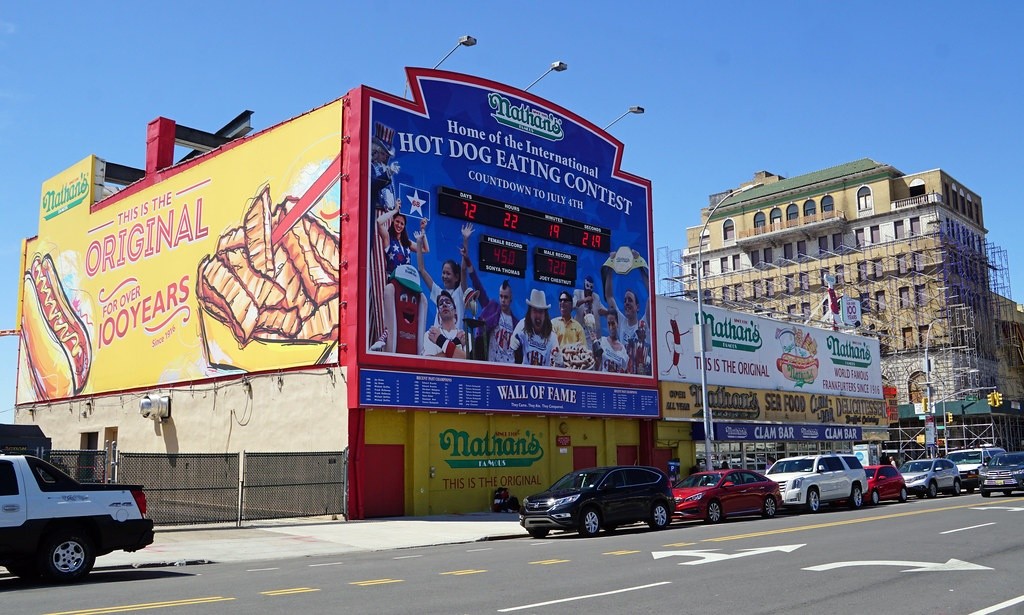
[
  {"x1": 896, "y1": 457, "x2": 963, "y2": 499},
  {"x1": 669, "y1": 469, "x2": 782, "y2": 525},
  {"x1": 829, "y1": 465, "x2": 908, "y2": 510}
]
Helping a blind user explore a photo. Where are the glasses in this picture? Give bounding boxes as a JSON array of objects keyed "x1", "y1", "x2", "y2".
[{"x1": 559, "y1": 298, "x2": 569, "y2": 303}]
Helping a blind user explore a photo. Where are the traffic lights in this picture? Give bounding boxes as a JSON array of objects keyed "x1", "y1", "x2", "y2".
[
  {"x1": 948, "y1": 412, "x2": 953, "y2": 424},
  {"x1": 921, "y1": 398, "x2": 928, "y2": 412},
  {"x1": 987, "y1": 392, "x2": 995, "y2": 406},
  {"x1": 938, "y1": 439, "x2": 945, "y2": 447},
  {"x1": 995, "y1": 392, "x2": 1004, "y2": 407}
]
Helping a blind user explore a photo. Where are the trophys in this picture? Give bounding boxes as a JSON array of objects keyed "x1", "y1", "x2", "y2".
[{"x1": 464, "y1": 298, "x2": 486, "y2": 360}]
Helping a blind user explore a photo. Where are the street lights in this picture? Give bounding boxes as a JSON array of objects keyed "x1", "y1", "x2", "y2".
[
  {"x1": 925, "y1": 318, "x2": 943, "y2": 459},
  {"x1": 697, "y1": 182, "x2": 765, "y2": 472}
]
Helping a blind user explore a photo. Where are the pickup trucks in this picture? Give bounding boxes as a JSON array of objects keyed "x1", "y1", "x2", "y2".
[{"x1": 0, "y1": 452, "x2": 155, "y2": 585}]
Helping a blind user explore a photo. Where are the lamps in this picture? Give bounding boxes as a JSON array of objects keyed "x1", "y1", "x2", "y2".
[
  {"x1": 524, "y1": 60, "x2": 568, "y2": 91},
  {"x1": 603, "y1": 105, "x2": 644, "y2": 130},
  {"x1": 433, "y1": 35, "x2": 477, "y2": 69}
]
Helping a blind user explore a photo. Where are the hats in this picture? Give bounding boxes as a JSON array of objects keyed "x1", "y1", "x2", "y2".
[
  {"x1": 390, "y1": 264, "x2": 423, "y2": 294},
  {"x1": 526, "y1": 290, "x2": 551, "y2": 309},
  {"x1": 462, "y1": 287, "x2": 480, "y2": 306}
]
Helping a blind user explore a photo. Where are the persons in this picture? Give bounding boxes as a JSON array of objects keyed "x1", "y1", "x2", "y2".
[
  {"x1": 369, "y1": 121, "x2": 652, "y2": 377},
  {"x1": 720, "y1": 461, "x2": 736, "y2": 482},
  {"x1": 936, "y1": 453, "x2": 946, "y2": 458},
  {"x1": 880, "y1": 452, "x2": 900, "y2": 470},
  {"x1": 689, "y1": 461, "x2": 704, "y2": 476}
]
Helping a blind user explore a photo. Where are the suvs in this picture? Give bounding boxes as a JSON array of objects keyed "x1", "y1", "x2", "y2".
[
  {"x1": 978, "y1": 452, "x2": 1024, "y2": 498},
  {"x1": 936, "y1": 444, "x2": 1007, "y2": 494},
  {"x1": 517, "y1": 465, "x2": 676, "y2": 540},
  {"x1": 764, "y1": 454, "x2": 869, "y2": 514}
]
